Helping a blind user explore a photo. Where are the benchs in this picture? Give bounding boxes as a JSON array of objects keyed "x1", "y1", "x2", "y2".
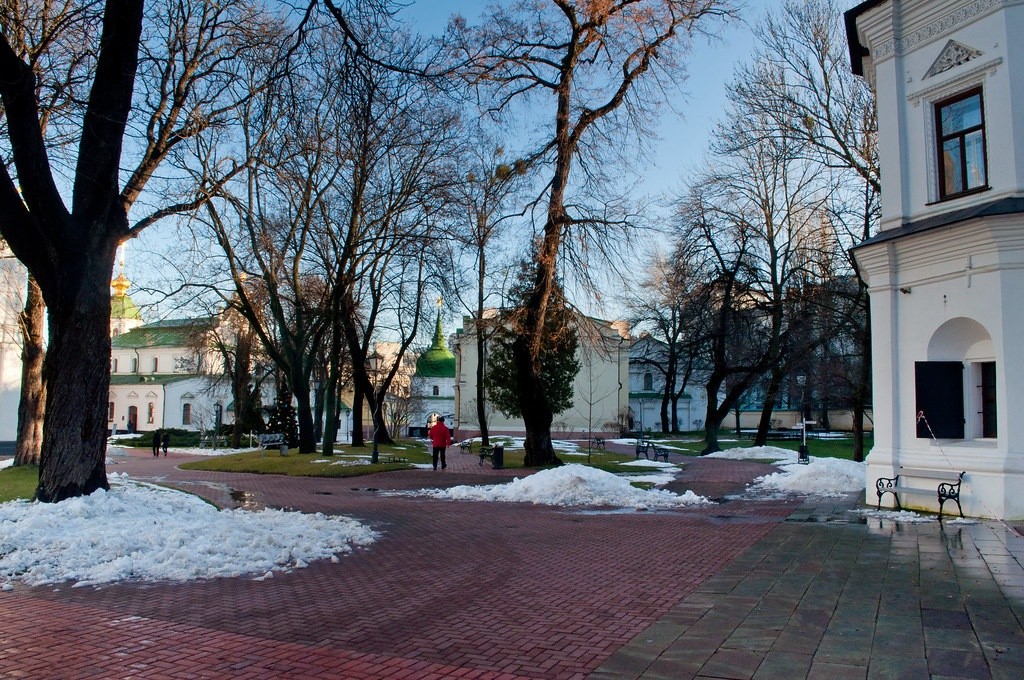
[
  {"x1": 876, "y1": 466, "x2": 967, "y2": 520},
  {"x1": 479, "y1": 444, "x2": 498, "y2": 466},
  {"x1": 460, "y1": 438, "x2": 473, "y2": 454},
  {"x1": 635, "y1": 438, "x2": 669, "y2": 463},
  {"x1": 592, "y1": 436, "x2": 606, "y2": 449}
]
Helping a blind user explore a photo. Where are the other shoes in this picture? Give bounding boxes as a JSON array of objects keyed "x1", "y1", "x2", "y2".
[
  {"x1": 442, "y1": 464, "x2": 447, "y2": 469},
  {"x1": 165, "y1": 454, "x2": 167, "y2": 456},
  {"x1": 434, "y1": 467, "x2": 437, "y2": 470}
]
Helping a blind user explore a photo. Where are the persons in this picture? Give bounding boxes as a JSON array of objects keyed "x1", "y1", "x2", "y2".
[
  {"x1": 429, "y1": 416, "x2": 451, "y2": 470},
  {"x1": 161, "y1": 432, "x2": 169, "y2": 456},
  {"x1": 152, "y1": 429, "x2": 161, "y2": 457}
]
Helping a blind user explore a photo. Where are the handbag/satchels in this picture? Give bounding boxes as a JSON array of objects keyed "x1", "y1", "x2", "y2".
[{"x1": 161, "y1": 442, "x2": 164, "y2": 446}]
